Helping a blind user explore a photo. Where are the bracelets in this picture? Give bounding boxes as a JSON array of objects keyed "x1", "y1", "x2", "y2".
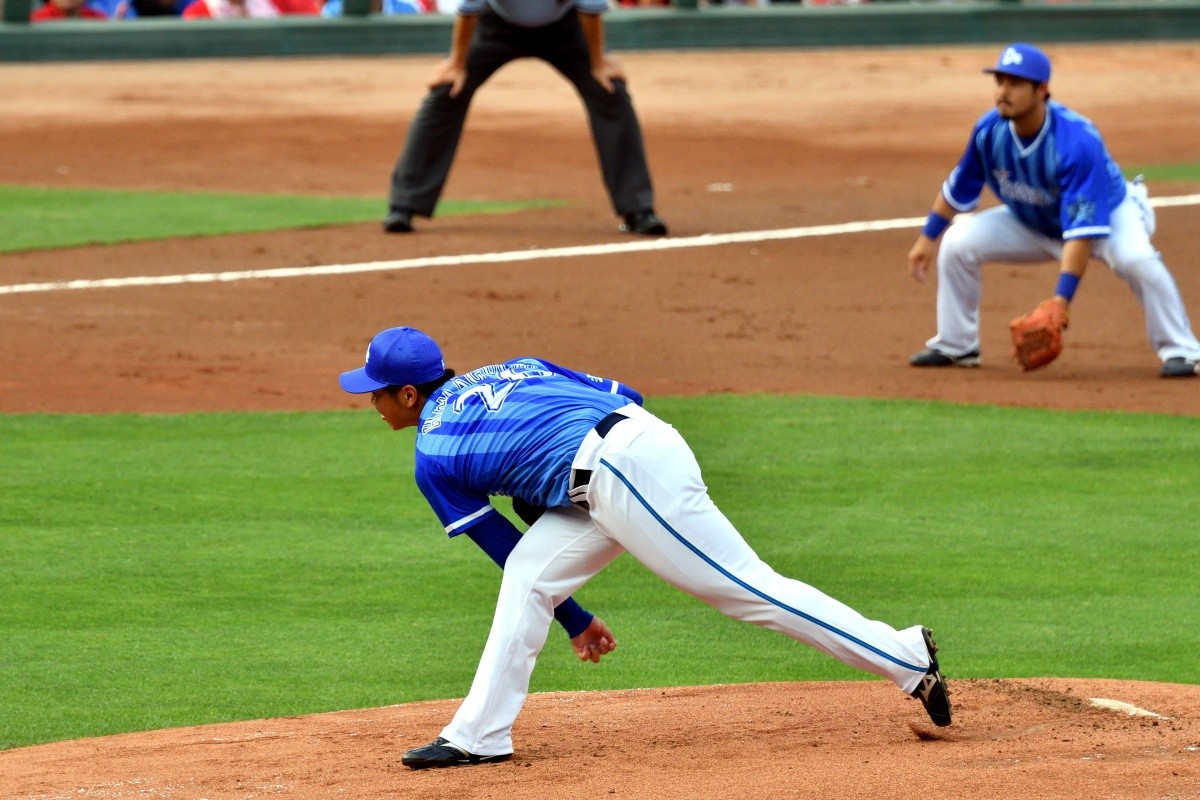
[
  {"x1": 1055, "y1": 273, "x2": 1080, "y2": 302},
  {"x1": 920, "y1": 213, "x2": 949, "y2": 240}
]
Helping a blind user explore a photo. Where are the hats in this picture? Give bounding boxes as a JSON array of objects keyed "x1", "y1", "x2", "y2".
[
  {"x1": 339, "y1": 326, "x2": 446, "y2": 394},
  {"x1": 982, "y1": 42, "x2": 1051, "y2": 84}
]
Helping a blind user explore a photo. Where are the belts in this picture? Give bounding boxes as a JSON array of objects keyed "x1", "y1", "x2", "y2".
[{"x1": 573, "y1": 411, "x2": 630, "y2": 505}]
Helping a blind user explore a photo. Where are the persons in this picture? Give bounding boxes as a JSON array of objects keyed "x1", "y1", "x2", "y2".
[
  {"x1": 340, "y1": 326, "x2": 953, "y2": 768},
  {"x1": 909, "y1": 42, "x2": 1200, "y2": 378},
  {"x1": 386, "y1": 0, "x2": 669, "y2": 237},
  {"x1": 30, "y1": 0, "x2": 424, "y2": 22}
]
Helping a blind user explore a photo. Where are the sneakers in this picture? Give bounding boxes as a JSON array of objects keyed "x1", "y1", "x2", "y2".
[
  {"x1": 912, "y1": 627, "x2": 952, "y2": 726},
  {"x1": 401, "y1": 736, "x2": 513, "y2": 767},
  {"x1": 907, "y1": 347, "x2": 983, "y2": 367},
  {"x1": 1158, "y1": 356, "x2": 1200, "y2": 377}
]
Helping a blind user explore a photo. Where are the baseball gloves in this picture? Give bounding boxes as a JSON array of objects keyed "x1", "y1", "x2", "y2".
[{"x1": 1010, "y1": 299, "x2": 1070, "y2": 371}]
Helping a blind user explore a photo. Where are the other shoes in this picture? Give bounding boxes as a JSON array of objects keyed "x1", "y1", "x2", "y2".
[
  {"x1": 382, "y1": 209, "x2": 411, "y2": 232},
  {"x1": 625, "y1": 208, "x2": 668, "y2": 235}
]
